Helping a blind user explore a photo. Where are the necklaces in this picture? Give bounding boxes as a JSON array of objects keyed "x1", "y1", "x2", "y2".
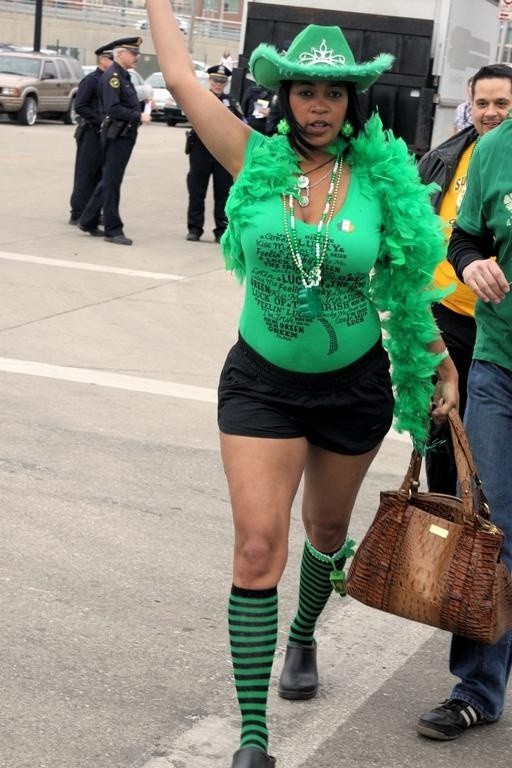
[{"x1": 282, "y1": 138, "x2": 342, "y2": 292}]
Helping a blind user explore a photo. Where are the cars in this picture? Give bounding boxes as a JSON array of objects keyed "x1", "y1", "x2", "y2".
[
  {"x1": 1, "y1": 42, "x2": 224, "y2": 124},
  {"x1": 137, "y1": 14, "x2": 189, "y2": 34}
]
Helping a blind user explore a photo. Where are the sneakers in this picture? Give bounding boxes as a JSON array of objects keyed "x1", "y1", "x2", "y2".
[{"x1": 416, "y1": 698, "x2": 484, "y2": 740}]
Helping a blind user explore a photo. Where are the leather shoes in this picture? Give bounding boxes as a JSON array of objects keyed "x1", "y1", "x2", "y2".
[
  {"x1": 229, "y1": 748, "x2": 278, "y2": 768},
  {"x1": 69, "y1": 215, "x2": 132, "y2": 246},
  {"x1": 279, "y1": 638, "x2": 319, "y2": 699},
  {"x1": 186, "y1": 231, "x2": 199, "y2": 241}
]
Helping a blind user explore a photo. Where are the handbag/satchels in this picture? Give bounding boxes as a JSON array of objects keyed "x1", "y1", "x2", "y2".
[{"x1": 343, "y1": 489, "x2": 511, "y2": 644}]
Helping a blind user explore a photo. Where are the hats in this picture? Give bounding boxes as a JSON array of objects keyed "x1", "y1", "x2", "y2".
[
  {"x1": 207, "y1": 64, "x2": 232, "y2": 82},
  {"x1": 95, "y1": 37, "x2": 143, "y2": 55},
  {"x1": 250, "y1": 24, "x2": 397, "y2": 93}
]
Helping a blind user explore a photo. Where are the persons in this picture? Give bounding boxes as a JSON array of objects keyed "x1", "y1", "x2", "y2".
[
  {"x1": 415, "y1": 109, "x2": 511, "y2": 743},
  {"x1": 450, "y1": 75, "x2": 476, "y2": 139},
  {"x1": 76, "y1": 37, "x2": 157, "y2": 246},
  {"x1": 184, "y1": 63, "x2": 239, "y2": 245},
  {"x1": 141, "y1": 0, "x2": 464, "y2": 768},
  {"x1": 68, "y1": 44, "x2": 115, "y2": 225},
  {"x1": 413, "y1": 63, "x2": 511, "y2": 495}
]
